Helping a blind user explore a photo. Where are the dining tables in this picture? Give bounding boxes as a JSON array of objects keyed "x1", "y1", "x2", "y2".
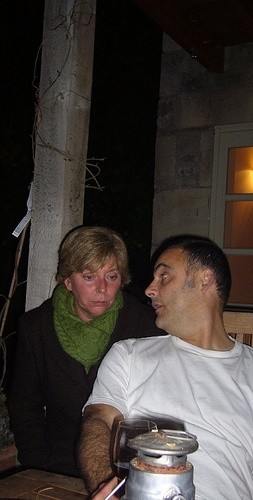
[{"x1": 0, "y1": 466, "x2": 91, "y2": 500}]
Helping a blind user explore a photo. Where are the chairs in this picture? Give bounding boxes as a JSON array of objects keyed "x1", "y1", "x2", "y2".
[{"x1": 220, "y1": 309, "x2": 253, "y2": 348}]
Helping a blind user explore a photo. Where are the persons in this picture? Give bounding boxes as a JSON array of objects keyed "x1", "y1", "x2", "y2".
[
  {"x1": 75, "y1": 232, "x2": 253, "y2": 500},
  {"x1": 2, "y1": 222, "x2": 178, "y2": 483}
]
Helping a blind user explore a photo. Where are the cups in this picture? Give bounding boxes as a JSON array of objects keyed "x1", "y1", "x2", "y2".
[{"x1": 111, "y1": 419, "x2": 158, "y2": 498}]
